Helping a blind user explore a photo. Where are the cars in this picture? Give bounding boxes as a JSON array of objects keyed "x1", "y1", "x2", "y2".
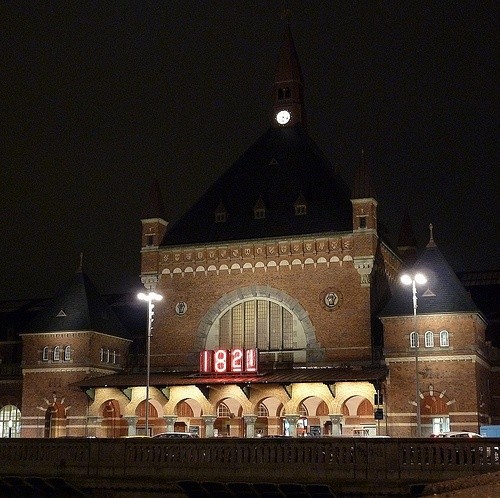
[
  {"x1": 431, "y1": 433, "x2": 499, "y2": 464},
  {"x1": 152, "y1": 432, "x2": 198, "y2": 440}
]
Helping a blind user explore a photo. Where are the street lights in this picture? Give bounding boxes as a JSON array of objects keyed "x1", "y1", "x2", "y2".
[
  {"x1": 399, "y1": 272, "x2": 429, "y2": 436},
  {"x1": 135, "y1": 292, "x2": 164, "y2": 437}
]
